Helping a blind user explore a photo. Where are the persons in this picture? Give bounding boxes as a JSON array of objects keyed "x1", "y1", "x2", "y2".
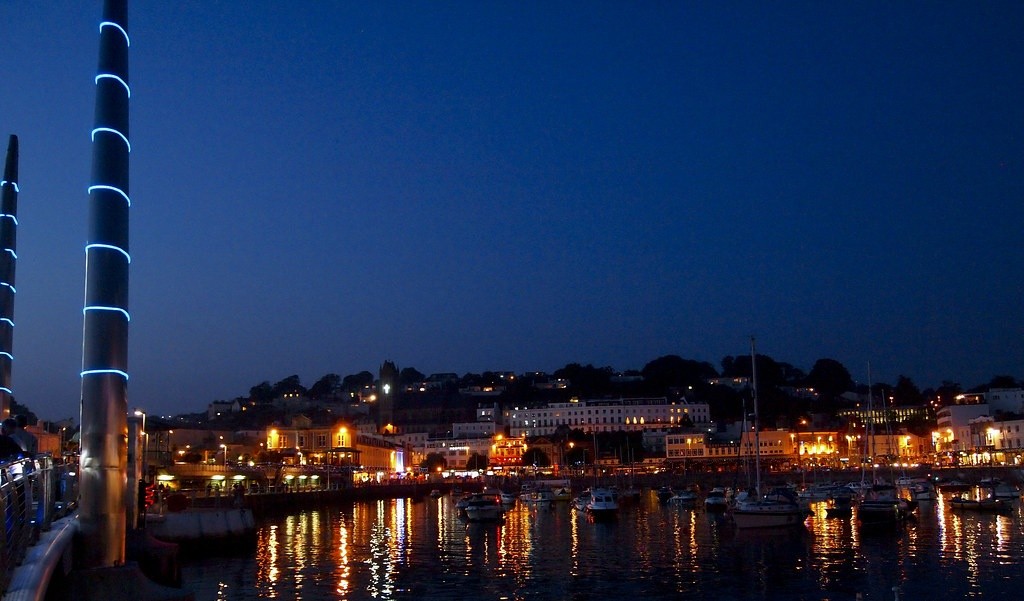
[
  {"x1": 9, "y1": 415, "x2": 38, "y2": 455},
  {"x1": 0, "y1": 419, "x2": 35, "y2": 469}
]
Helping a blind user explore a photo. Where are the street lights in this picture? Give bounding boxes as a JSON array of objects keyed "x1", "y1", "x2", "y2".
[{"x1": 220, "y1": 443, "x2": 227, "y2": 470}]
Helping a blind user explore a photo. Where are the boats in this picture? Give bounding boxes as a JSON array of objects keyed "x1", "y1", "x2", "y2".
[{"x1": 430, "y1": 331, "x2": 1023, "y2": 531}]
